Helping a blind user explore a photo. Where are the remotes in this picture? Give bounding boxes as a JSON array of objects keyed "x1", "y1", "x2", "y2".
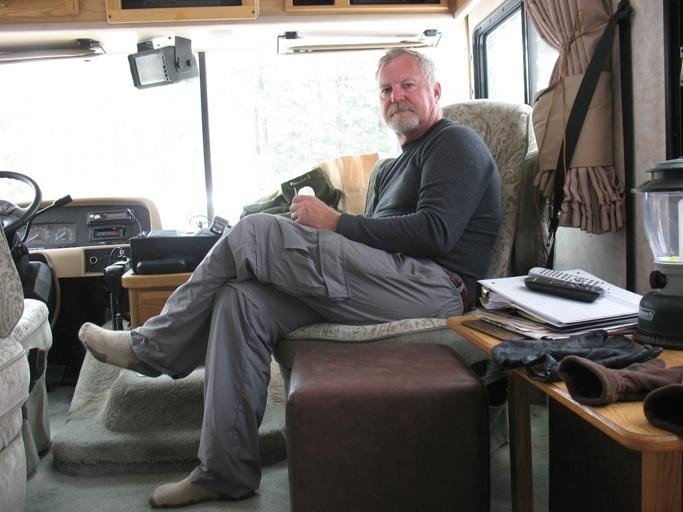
[
  {"x1": 298, "y1": 187, "x2": 315, "y2": 196},
  {"x1": 528, "y1": 266, "x2": 610, "y2": 291},
  {"x1": 524, "y1": 276, "x2": 603, "y2": 302},
  {"x1": 210, "y1": 216, "x2": 229, "y2": 235}
]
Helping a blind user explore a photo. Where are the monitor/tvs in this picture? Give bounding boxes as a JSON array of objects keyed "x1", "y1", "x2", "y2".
[{"x1": 128, "y1": 47, "x2": 198, "y2": 89}]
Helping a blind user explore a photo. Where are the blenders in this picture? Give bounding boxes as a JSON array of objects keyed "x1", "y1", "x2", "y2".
[{"x1": 629, "y1": 155, "x2": 683, "y2": 350}]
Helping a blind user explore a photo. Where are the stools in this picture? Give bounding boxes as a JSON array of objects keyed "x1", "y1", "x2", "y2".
[{"x1": 285, "y1": 343, "x2": 490, "y2": 512}]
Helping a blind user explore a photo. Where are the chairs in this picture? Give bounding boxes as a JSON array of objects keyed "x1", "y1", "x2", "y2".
[
  {"x1": 0, "y1": 222, "x2": 54, "y2": 512},
  {"x1": 273, "y1": 99, "x2": 552, "y2": 376}
]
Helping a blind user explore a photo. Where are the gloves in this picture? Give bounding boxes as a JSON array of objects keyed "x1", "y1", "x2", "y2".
[
  {"x1": 560, "y1": 354, "x2": 683, "y2": 441},
  {"x1": 493, "y1": 330, "x2": 663, "y2": 383}
]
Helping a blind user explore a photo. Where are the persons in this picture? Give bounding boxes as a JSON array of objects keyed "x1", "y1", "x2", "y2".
[{"x1": 78, "y1": 47, "x2": 503, "y2": 508}]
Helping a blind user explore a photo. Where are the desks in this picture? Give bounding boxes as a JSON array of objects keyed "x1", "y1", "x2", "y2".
[{"x1": 446, "y1": 269, "x2": 683, "y2": 512}]
